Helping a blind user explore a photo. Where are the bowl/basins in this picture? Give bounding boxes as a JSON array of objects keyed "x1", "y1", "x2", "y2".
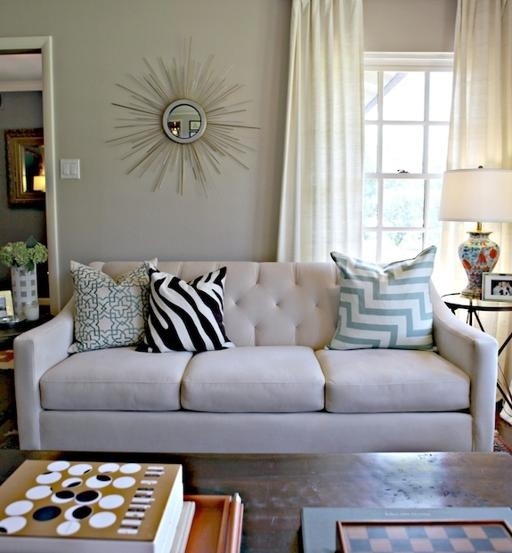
[{"x1": 0, "y1": 315, "x2": 27, "y2": 328}]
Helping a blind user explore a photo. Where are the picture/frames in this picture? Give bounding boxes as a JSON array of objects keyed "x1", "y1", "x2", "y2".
[
  {"x1": 4, "y1": 127, "x2": 46, "y2": 209},
  {"x1": 482, "y1": 271, "x2": 512, "y2": 301}
]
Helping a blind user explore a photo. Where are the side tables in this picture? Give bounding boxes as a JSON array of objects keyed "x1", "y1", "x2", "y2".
[{"x1": 441, "y1": 293, "x2": 512, "y2": 417}]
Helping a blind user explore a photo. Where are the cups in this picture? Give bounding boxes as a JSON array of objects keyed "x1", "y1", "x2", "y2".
[{"x1": 23, "y1": 301, "x2": 40, "y2": 320}]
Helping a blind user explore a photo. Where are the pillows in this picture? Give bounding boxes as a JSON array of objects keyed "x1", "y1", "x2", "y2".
[
  {"x1": 66, "y1": 256, "x2": 237, "y2": 353},
  {"x1": 324, "y1": 245, "x2": 439, "y2": 353}
]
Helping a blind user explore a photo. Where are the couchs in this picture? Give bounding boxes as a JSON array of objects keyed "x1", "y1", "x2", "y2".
[{"x1": 14, "y1": 259, "x2": 498, "y2": 453}]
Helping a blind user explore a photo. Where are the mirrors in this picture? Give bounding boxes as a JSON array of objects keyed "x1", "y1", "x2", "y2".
[{"x1": 162, "y1": 97, "x2": 208, "y2": 144}]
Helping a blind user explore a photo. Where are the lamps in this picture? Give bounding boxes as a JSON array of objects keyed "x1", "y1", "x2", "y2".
[{"x1": 438, "y1": 164, "x2": 512, "y2": 299}]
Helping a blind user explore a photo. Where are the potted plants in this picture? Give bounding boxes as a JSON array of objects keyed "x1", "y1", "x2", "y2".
[{"x1": 2, "y1": 235, "x2": 49, "y2": 321}]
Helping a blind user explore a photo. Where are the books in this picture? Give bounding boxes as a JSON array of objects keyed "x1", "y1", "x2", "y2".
[
  {"x1": 0, "y1": 348, "x2": 16, "y2": 370},
  {"x1": 0, "y1": 461, "x2": 199, "y2": 553}
]
